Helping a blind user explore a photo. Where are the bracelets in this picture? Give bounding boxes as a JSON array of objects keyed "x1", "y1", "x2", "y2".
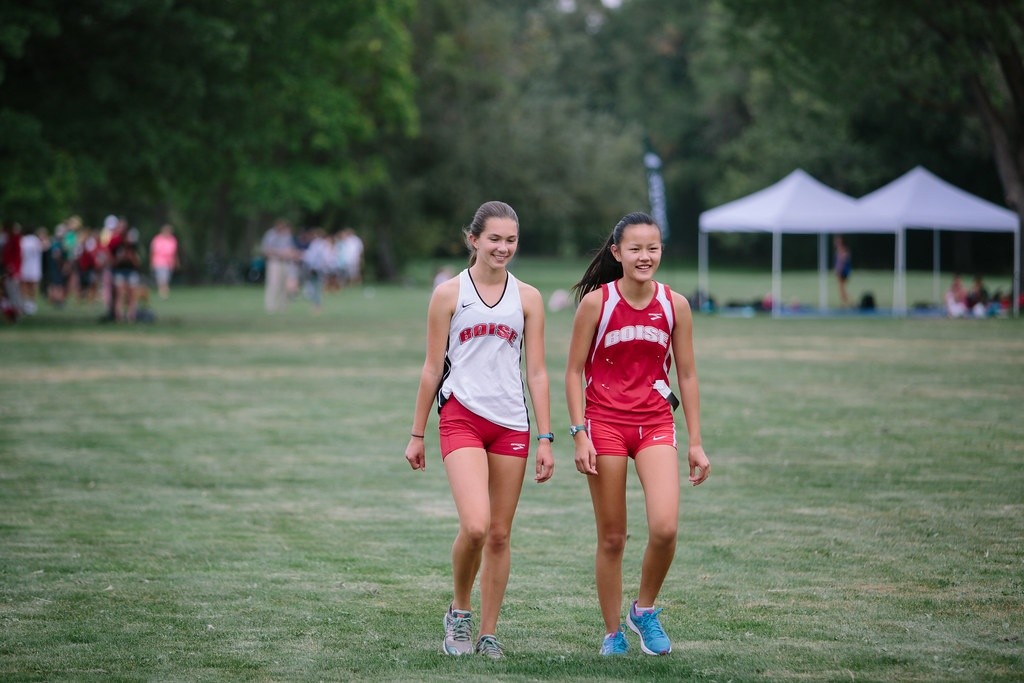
[{"x1": 411, "y1": 434, "x2": 425, "y2": 440}]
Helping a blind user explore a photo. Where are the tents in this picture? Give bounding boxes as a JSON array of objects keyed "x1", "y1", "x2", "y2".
[
  {"x1": 863, "y1": 166, "x2": 1022, "y2": 318},
  {"x1": 697, "y1": 165, "x2": 903, "y2": 318}
]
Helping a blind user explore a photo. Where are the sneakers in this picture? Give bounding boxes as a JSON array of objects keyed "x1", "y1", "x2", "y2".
[
  {"x1": 476, "y1": 634, "x2": 507, "y2": 660},
  {"x1": 443, "y1": 604, "x2": 473, "y2": 655},
  {"x1": 599, "y1": 632, "x2": 628, "y2": 655},
  {"x1": 626, "y1": 599, "x2": 672, "y2": 656}
]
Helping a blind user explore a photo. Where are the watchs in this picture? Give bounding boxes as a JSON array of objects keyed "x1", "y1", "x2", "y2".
[
  {"x1": 537, "y1": 432, "x2": 554, "y2": 443},
  {"x1": 570, "y1": 424, "x2": 586, "y2": 437}
]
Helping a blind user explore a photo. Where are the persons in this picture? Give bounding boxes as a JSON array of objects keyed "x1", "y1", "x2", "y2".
[
  {"x1": 406, "y1": 199, "x2": 556, "y2": 660},
  {"x1": 565, "y1": 211, "x2": 710, "y2": 660},
  {"x1": 948, "y1": 271, "x2": 991, "y2": 319},
  {"x1": 0, "y1": 209, "x2": 570, "y2": 328},
  {"x1": 833, "y1": 234, "x2": 852, "y2": 307}
]
